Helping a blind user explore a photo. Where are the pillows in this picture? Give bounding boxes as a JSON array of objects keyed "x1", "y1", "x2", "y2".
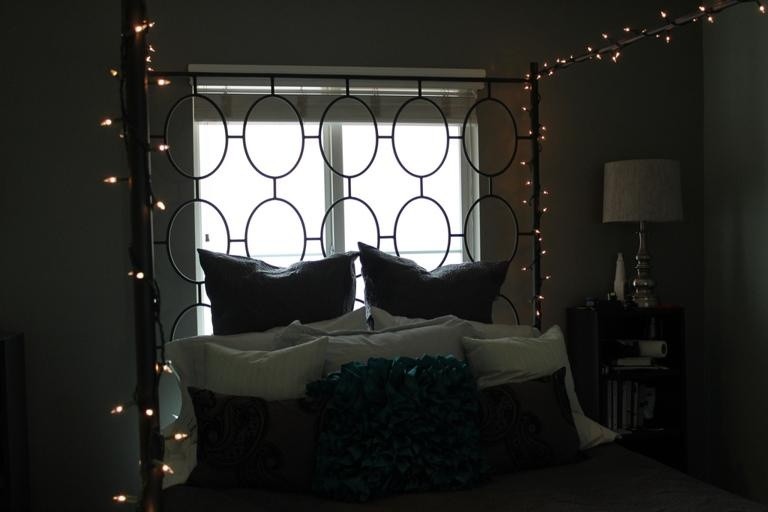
[
  {"x1": 482, "y1": 366, "x2": 586, "y2": 471},
  {"x1": 197, "y1": 246, "x2": 360, "y2": 336},
  {"x1": 305, "y1": 354, "x2": 492, "y2": 490},
  {"x1": 202, "y1": 336, "x2": 330, "y2": 400},
  {"x1": 185, "y1": 385, "x2": 311, "y2": 487},
  {"x1": 371, "y1": 305, "x2": 541, "y2": 338},
  {"x1": 457, "y1": 323, "x2": 622, "y2": 452},
  {"x1": 169, "y1": 308, "x2": 368, "y2": 446},
  {"x1": 359, "y1": 242, "x2": 509, "y2": 324}
]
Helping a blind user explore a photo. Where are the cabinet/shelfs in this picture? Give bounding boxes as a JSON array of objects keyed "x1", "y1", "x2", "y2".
[{"x1": 564, "y1": 305, "x2": 690, "y2": 471}]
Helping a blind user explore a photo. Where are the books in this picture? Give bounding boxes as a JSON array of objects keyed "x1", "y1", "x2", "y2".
[{"x1": 598, "y1": 354, "x2": 657, "y2": 432}]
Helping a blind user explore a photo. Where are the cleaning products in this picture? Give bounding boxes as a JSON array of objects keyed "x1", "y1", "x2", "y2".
[{"x1": 612, "y1": 252, "x2": 629, "y2": 306}]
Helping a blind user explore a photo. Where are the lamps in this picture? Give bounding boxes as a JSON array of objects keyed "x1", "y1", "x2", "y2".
[{"x1": 601, "y1": 158, "x2": 687, "y2": 310}]
